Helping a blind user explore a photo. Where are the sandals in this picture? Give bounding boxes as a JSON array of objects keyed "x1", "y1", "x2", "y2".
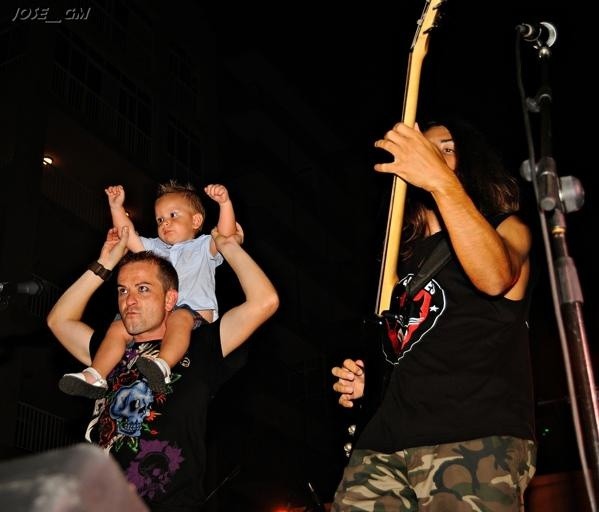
[
  {"x1": 59, "y1": 367, "x2": 109, "y2": 399},
  {"x1": 136, "y1": 356, "x2": 170, "y2": 396}
]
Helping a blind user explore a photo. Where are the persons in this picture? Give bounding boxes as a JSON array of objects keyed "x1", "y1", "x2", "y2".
[
  {"x1": 59, "y1": 182, "x2": 238, "y2": 399},
  {"x1": 46, "y1": 221, "x2": 280, "y2": 511},
  {"x1": 329, "y1": 117, "x2": 537, "y2": 512}
]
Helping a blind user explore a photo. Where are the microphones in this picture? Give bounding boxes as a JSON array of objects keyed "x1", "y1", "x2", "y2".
[
  {"x1": 0, "y1": 278, "x2": 52, "y2": 298},
  {"x1": 514, "y1": 21, "x2": 559, "y2": 50}
]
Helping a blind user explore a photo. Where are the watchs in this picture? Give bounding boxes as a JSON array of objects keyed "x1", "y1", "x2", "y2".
[{"x1": 88, "y1": 261, "x2": 111, "y2": 282}]
